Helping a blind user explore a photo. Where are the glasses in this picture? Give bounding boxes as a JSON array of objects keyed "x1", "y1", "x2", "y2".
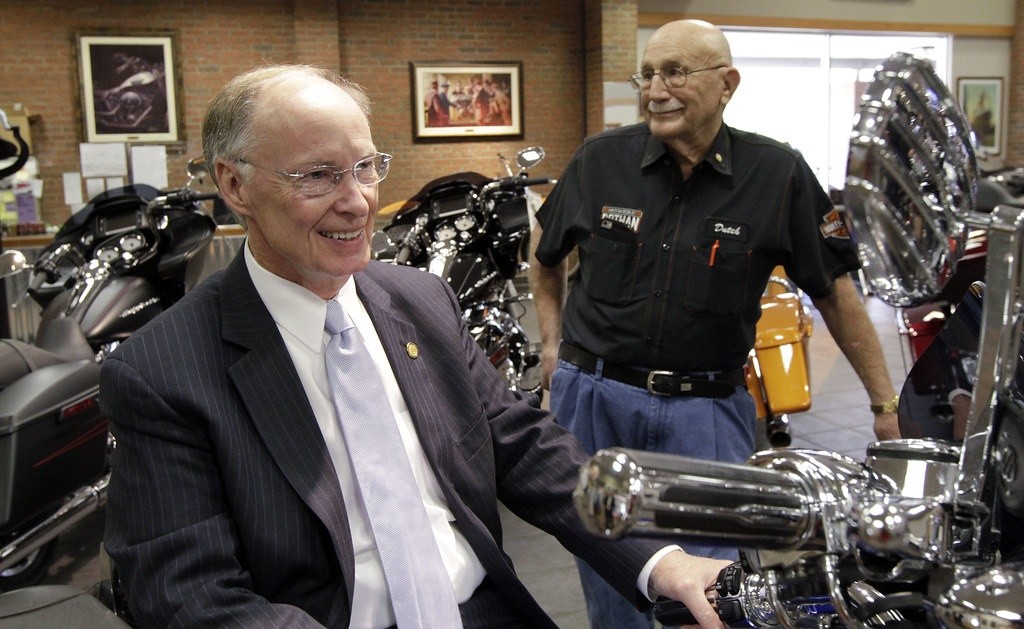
[
  {"x1": 628, "y1": 65, "x2": 727, "y2": 93},
  {"x1": 237, "y1": 143, "x2": 393, "y2": 195}
]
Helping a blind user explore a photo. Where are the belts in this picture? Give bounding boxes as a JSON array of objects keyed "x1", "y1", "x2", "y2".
[{"x1": 558, "y1": 341, "x2": 745, "y2": 400}]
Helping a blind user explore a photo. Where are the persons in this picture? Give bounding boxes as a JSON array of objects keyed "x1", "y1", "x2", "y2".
[
  {"x1": 529, "y1": 19, "x2": 900, "y2": 629},
  {"x1": 898, "y1": 276, "x2": 986, "y2": 444},
  {"x1": 101, "y1": 62, "x2": 735, "y2": 628}
]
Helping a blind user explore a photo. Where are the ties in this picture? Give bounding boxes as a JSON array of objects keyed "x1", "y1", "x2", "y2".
[{"x1": 324, "y1": 302, "x2": 463, "y2": 629}]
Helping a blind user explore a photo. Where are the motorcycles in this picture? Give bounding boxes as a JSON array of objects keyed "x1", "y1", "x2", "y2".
[
  {"x1": 573, "y1": 51, "x2": 1024, "y2": 629},
  {"x1": 370, "y1": 146, "x2": 558, "y2": 405},
  {"x1": 0, "y1": 155, "x2": 219, "y2": 601}
]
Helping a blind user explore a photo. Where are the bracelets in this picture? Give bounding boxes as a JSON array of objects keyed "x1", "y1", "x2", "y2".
[{"x1": 871, "y1": 396, "x2": 899, "y2": 414}]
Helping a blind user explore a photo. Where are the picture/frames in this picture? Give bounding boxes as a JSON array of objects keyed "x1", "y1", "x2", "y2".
[
  {"x1": 409, "y1": 60, "x2": 526, "y2": 144},
  {"x1": 956, "y1": 76, "x2": 1006, "y2": 156},
  {"x1": 68, "y1": 26, "x2": 188, "y2": 155}
]
